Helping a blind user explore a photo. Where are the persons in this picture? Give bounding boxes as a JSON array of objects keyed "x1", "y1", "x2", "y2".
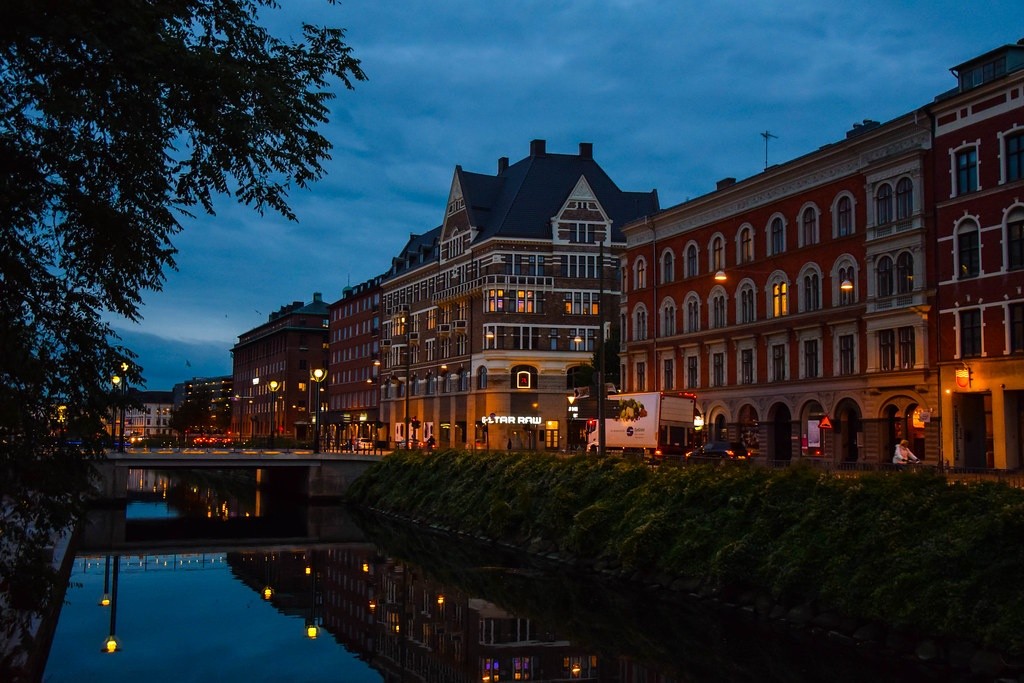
[
  {"x1": 427, "y1": 435, "x2": 435, "y2": 450},
  {"x1": 892, "y1": 440, "x2": 922, "y2": 465},
  {"x1": 507, "y1": 438, "x2": 512, "y2": 455}
]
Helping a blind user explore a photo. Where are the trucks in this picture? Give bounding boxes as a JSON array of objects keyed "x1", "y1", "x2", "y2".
[{"x1": 585, "y1": 390, "x2": 697, "y2": 458}]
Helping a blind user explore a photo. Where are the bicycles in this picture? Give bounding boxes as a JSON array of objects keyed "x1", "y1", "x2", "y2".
[{"x1": 898, "y1": 459, "x2": 920, "y2": 473}]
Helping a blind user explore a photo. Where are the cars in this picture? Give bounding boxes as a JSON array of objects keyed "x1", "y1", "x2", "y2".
[
  {"x1": 353, "y1": 437, "x2": 373, "y2": 451},
  {"x1": 397, "y1": 438, "x2": 423, "y2": 451},
  {"x1": 684, "y1": 440, "x2": 750, "y2": 468}
]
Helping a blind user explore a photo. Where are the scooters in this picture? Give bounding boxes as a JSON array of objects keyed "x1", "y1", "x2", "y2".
[{"x1": 427, "y1": 441, "x2": 435, "y2": 455}]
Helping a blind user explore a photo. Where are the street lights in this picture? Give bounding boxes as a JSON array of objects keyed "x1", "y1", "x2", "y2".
[
  {"x1": 111, "y1": 375, "x2": 121, "y2": 453},
  {"x1": 306, "y1": 551, "x2": 319, "y2": 639},
  {"x1": 120, "y1": 361, "x2": 129, "y2": 455},
  {"x1": 99, "y1": 555, "x2": 111, "y2": 608},
  {"x1": 266, "y1": 378, "x2": 284, "y2": 451},
  {"x1": 259, "y1": 551, "x2": 277, "y2": 602},
  {"x1": 101, "y1": 553, "x2": 125, "y2": 655},
  {"x1": 309, "y1": 366, "x2": 329, "y2": 454}
]
